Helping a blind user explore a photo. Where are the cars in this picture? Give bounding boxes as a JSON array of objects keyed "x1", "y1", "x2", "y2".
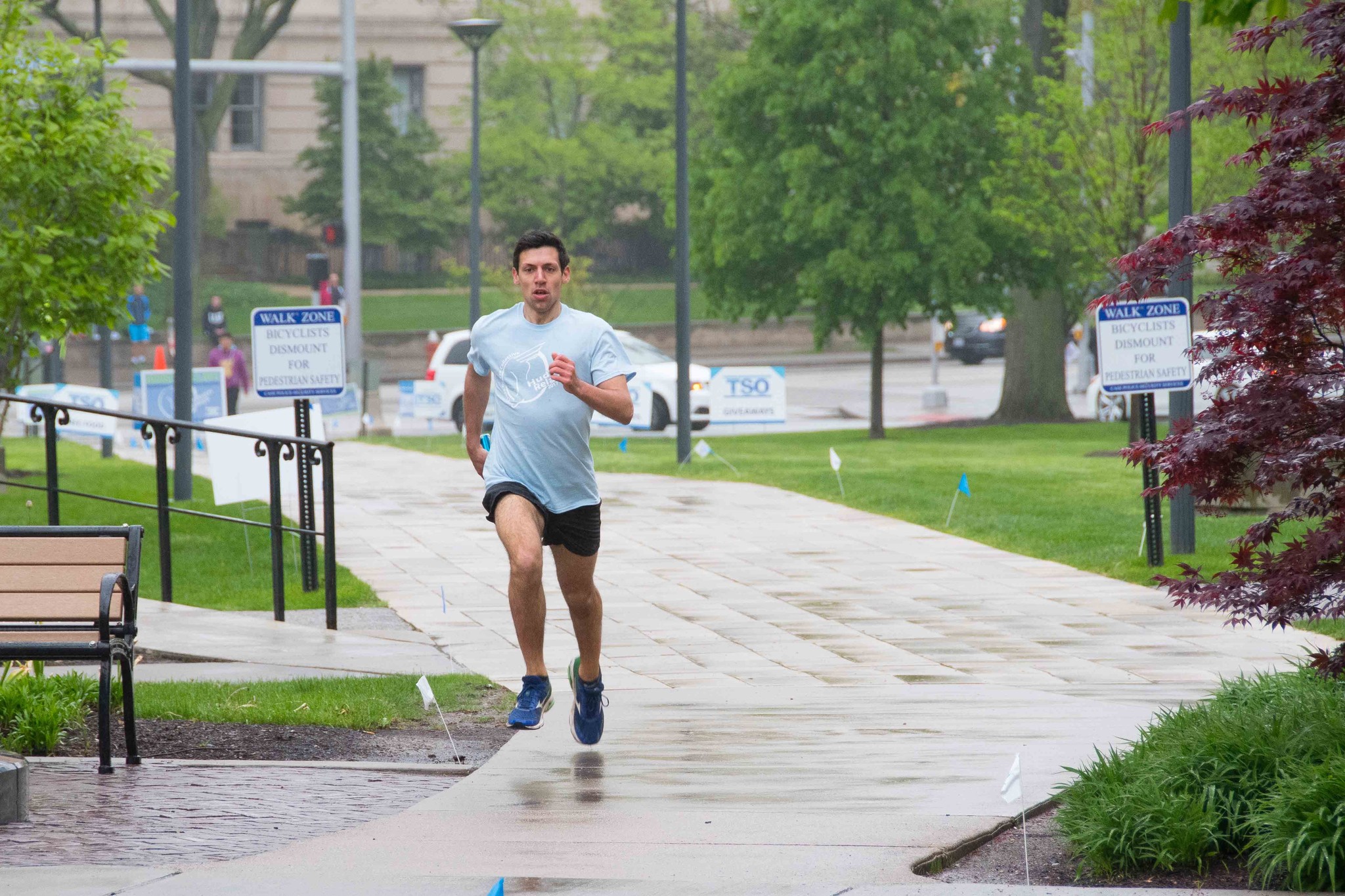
[
  {"x1": 425, "y1": 330, "x2": 709, "y2": 432},
  {"x1": 944, "y1": 270, "x2": 1166, "y2": 367},
  {"x1": 1085, "y1": 330, "x2": 1342, "y2": 422}
]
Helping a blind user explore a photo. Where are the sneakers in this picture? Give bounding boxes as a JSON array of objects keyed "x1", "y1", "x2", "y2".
[
  {"x1": 506, "y1": 674, "x2": 555, "y2": 730},
  {"x1": 568, "y1": 656, "x2": 609, "y2": 744}
]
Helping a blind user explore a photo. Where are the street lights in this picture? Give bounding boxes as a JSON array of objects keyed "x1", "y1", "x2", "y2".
[{"x1": 447, "y1": 18, "x2": 501, "y2": 330}]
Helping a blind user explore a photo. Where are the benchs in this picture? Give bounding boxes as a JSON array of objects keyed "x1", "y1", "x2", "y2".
[{"x1": 0, "y1": 525, "x2": 144, "y2": 775}]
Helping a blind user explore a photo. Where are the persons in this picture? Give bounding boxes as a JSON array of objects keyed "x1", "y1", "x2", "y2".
[
  {"x1": 208, "y1": 332, "x2": 250, "y2": 415},
  {"x1": 325, "y1": 273, "x2": 345, "y2": 318},
  {"x1": 202, "y1": 295, "x2": 226, "y2": 348},
  {"x1": 462, "y1": 229, "x2": 637, "y2": 745},
  {"x1": 126, "y1": 283, "x2": 151, "y2": 365}
]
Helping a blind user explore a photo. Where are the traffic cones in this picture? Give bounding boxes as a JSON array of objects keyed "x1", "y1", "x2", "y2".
[{"x1": 153, "y1": 344, "x2": 167, "y2": 371}]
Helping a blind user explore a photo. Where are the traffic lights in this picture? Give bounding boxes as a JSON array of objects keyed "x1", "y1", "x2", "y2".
[{"x1": 320, "y1": 223, "x2": 343, "y2": 246}]
